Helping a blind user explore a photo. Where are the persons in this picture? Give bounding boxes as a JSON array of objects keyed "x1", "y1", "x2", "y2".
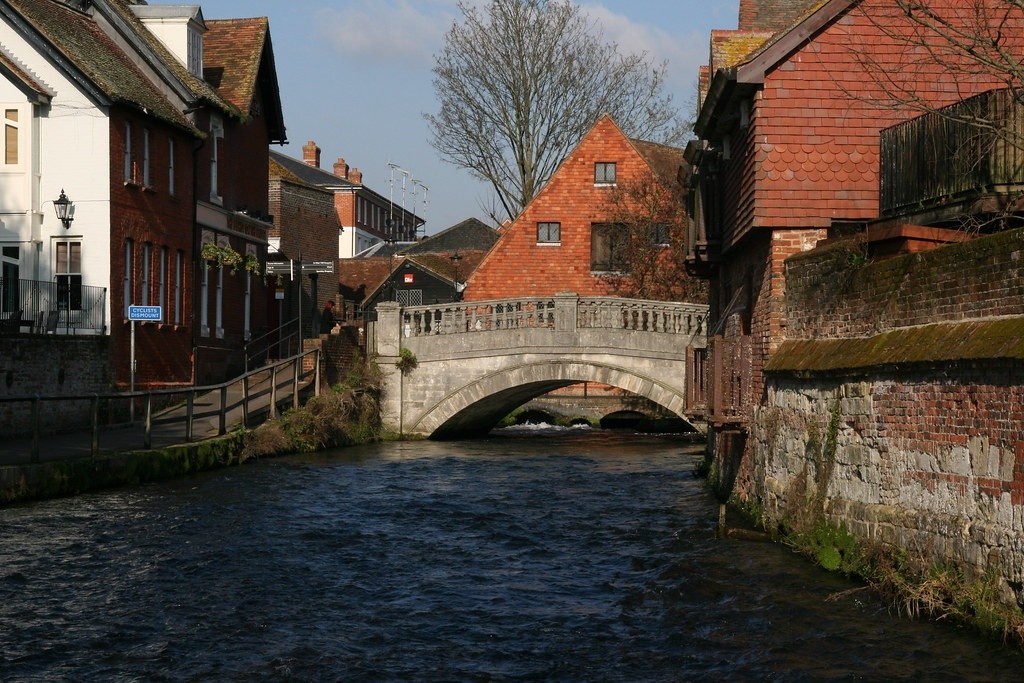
[{"x1": 321, "y1": 301, "x2": 336, "y2": 334}]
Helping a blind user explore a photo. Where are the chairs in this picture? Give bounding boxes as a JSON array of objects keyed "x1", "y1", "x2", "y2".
[{"x1": 0, "y1": 307, "x2": 60, "y2": 334}]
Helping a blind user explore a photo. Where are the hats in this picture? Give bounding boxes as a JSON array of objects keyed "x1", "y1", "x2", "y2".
[{"x1": 328, "y1": 300, "x2": 336, "y2": 306}]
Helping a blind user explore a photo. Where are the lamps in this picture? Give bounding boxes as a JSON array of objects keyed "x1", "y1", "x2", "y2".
[{"x1": 53, "y1": 188, "x2": 74, "y2": 229}]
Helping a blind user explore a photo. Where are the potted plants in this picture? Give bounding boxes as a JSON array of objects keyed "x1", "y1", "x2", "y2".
[{"x1": 201, "y1": 242, "x2": 262, "y2": 276}]
